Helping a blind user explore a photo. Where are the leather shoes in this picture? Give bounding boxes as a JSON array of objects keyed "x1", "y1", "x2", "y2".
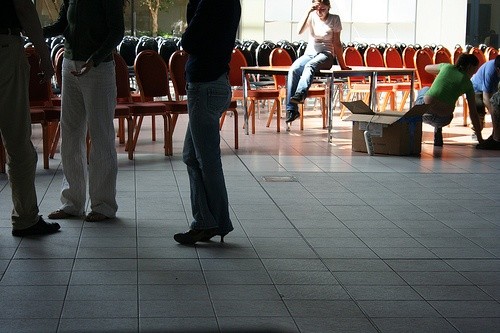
[
  {"x1": 290, "y1": 93, "x2": 304, "y2": 104},
  {"x1": 285, "y1": 111, "x2": 300, "y2": 123}
]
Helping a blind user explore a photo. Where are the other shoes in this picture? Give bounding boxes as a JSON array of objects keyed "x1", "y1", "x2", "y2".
[
  {"x1": 476, "y1": 135, "x2": 500, "y2": 150},
  {"x1": 434, "y1": 137, "x2": 443, "y2": 145}
]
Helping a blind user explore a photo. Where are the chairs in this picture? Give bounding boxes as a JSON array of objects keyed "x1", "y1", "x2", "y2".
[{"x1": 0, "y1": 35, "x2": 500, "y2": 173}]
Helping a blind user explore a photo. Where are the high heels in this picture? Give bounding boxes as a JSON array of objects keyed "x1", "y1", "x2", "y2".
[
  {"x1": 173, "y1": 227, "x2": 225, "y2": 247},
  {"x1": 198, "y1": 224, "x2": 234, "y2": 242}
]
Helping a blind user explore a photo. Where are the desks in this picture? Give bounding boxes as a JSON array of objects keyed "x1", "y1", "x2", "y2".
[{"x1": 240, "y1": 64, "x2": 418, "y2": 145}]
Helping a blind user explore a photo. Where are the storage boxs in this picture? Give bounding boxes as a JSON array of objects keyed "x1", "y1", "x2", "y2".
[{"x1": 341, "y1": 99, "x2": 427, "y2": 156}]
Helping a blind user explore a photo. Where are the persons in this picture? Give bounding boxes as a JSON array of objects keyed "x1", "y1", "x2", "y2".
[
  {"x1": 0, "y1": 0, "x2": 60, "y2": 238},
  {"x1": 464, "y1": 55, "x2": 500, "y2": 131},
  {"x1": 284, "y1": 0, "x2": 352, "y2": 122},
  {"x1": 173, "y1": 0, "x2": 241, "y2": 245},
  {"x1": 475, "y1": 83, "x2": 500, "y2": 151},
  {"x1": 45, "y1": 0, "x2": 126, "y2": 223},
  {"x1": 414, "y1": 53, "x2": 486, "y2": 146}
]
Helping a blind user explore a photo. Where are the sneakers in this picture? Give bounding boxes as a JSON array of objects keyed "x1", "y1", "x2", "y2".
[
  {"x1": 47, "y1": 209, "x2": 86, "y2": 219},
  {"x1": 12, "y1": 216, "x2": 61, "y2": 236},
  {"x1": 86, "y1": 211, "x2": 109, "y2": 221}
]
